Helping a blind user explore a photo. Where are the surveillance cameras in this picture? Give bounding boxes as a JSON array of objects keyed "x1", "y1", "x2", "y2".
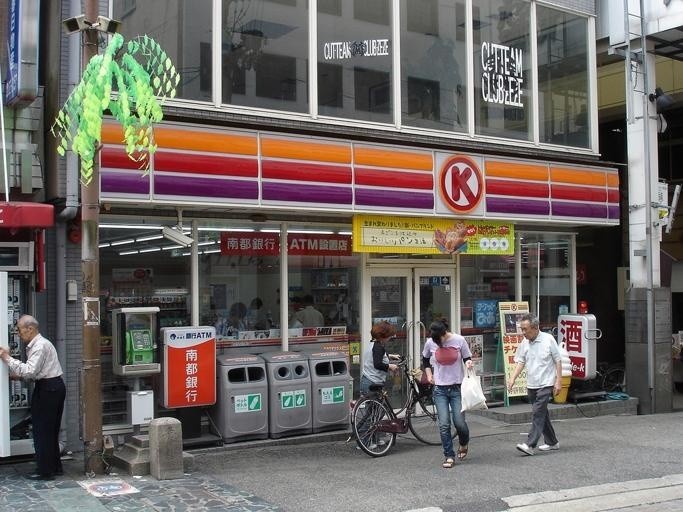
[
  {"x1": 61, "y1": 14, "x2": 89, "y2": 35},
  {"x1": 93, "y1": 15, "x2": 122, "y2": 34}
]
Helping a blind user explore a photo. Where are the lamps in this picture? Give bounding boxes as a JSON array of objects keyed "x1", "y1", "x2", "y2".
[
  {"x1": 649, "y1": 87, "x2": 663, "y2": 101},
  {"x1": 98, "y1": 224, "x2": 353, "y2": 256}
]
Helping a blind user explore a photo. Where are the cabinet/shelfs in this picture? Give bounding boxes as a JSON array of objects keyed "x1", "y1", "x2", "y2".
[
  {"x1": 312, "y1": 268, "x2": 349, "y2": 305},
  {"x1": 106, "y1": 295, "x2": 187, "y2": 340},
  {"x1": 478, "y1": 371, "x2": 506, "y2": 408}
]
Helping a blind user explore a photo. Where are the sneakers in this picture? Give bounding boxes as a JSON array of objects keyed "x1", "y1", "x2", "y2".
[
  {"x1": 354, "y1": 441, "x2": 377, "y2": 450},
  {"x1": 373, "y1": 440, "x2": 386, "y2": 446},
  {"x1": 515, "y1": 442, "x2": 535, "y2": 456},
  {"x1": 538, "y1": 441, "x2": 559, "y2": 451}
]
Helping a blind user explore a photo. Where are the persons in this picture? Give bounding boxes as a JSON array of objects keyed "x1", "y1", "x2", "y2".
[
  {"x1": 242, "y1": 297, "x2": 264, "y2": 330},
  {"x1": 0, "y1": 314, "x2": 67, "y2": 482},
  {"x1": 507, "y1": 313, "x2": 561, "y2": 455},
  {"x1": 357, "y1": 322, "x2": 401, "y2": 451},
  {"x1": 289, "y1": 295, "x2": 325, "y2": 327},
  {"x1": 422, "y1": 317, "x2": 474, "y2": 468},
  {"x1": 214, "y1": 302, "x2": 246, "y2": 336}
]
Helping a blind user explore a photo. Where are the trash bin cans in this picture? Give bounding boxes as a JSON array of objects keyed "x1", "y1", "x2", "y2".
[
  {"x1": 211, "y1": 354, "x2": 269, "y2": 443},
  {"x1": 258, "y1": 351, "x2": 312, "y2": 439},
  {"x1": 301, "y1": 350, "x2": 350, "y2": 434}
]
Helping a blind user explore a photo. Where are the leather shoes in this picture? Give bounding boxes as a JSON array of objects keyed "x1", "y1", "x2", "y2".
[
  {"x1": 24, "y1": 471, "x2": 57, "y2": 480},
  {"x1": 35, "y1": 466, "x2": 63, "y2": 475}
]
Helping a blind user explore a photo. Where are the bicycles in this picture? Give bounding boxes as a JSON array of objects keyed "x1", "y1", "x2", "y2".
[
  {"x1": 589, "y1": 360, "x2": 626, "y2": 393},
  {"x1": 350, "y1": 354, "x2": 459, "y2": 458}
]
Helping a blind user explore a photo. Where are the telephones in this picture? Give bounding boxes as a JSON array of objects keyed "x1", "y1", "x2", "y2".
[{"x1": 126, "y1": 325, "x2": 153, "y2": 363}]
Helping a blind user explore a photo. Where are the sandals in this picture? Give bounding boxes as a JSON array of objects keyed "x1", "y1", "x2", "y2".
[
  {"x1": 456, "y1": 443, "x2": 469, "y2": 460},
  {"x1": 442, "y1": 455, "x2": 455, "y2": 468}
]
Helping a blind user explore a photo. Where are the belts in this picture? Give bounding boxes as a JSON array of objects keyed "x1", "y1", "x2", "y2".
[{"x1": 34, "y1": 376, "x2": 61, "y2": 383}]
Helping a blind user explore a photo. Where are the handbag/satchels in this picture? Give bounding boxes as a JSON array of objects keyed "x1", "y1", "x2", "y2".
[
  {"x1": 411, "y1": 368, "x2": 432, "y2": 385},
  {"x1": 460, "y1": 374, "x2": 489, "y2": 413}
]
